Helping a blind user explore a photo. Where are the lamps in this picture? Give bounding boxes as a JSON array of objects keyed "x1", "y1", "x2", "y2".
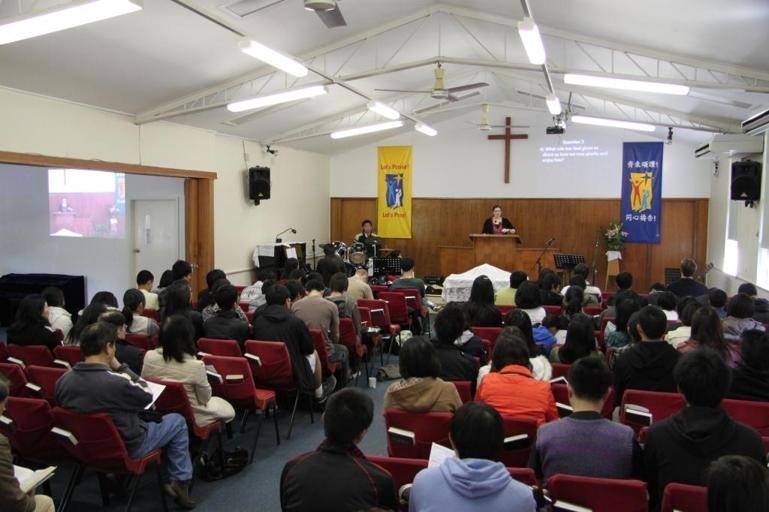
[
  {"x1": 329, "y1": 101, "x2": 437, "y2": 140},
  {"x1": 239, "y1": 36, "x2": 308, "y2": 77},
  {"x1": 516, "y1": 17, "x2": 545, "y2": 64},
  {"x1": 546, "y1": 95, "x2": 564, "y2": 117},
  {"x1": 0, "y1": 1, "x2": 145, "y2": 44},
  {"x1": 226, "y1": 83, "x2": 329, "y2": 116},
  {"x1": 571, "y1": 114, "x2": 657, "y2": 134},
  {"x1": 562, "y1": 69, "x2": 693, "y2": 95}
]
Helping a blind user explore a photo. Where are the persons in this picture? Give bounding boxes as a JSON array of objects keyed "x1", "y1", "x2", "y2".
[
  {"x1": 481, "y1": 205, "x2": 516, "y2": 235},
  {"x1": 385, "y1": 256, "x2": 769, "y2": 511},
  {"x1": 1, "y1": 242, "x2": 425, "y2": 510},
  {"x1": 353, "y1": 219, "x2": 381, "y2": 259}
]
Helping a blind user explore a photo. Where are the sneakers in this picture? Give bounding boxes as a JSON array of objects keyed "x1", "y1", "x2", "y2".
[
  {"x1": 315, "y1": 376, "x2": 337, "y2": 403},
  {"x1": 164, "y1": 480, "x2": 195, "y2": 507}
]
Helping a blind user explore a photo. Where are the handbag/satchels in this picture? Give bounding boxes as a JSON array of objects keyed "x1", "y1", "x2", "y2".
[{"x1": 194, "y1": 448, "x2": 248, "y2": 481}]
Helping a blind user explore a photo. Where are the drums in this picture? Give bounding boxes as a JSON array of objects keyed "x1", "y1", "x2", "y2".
[
  {"x1": 379, "y1": 249, "x2": 394, "y2": 258},
  {"x1": 348, "y1": 242, "x2": 368, "y2": 265},
  {"x1": 331, "y1": 241, "x2": 348, "y2": 260}
]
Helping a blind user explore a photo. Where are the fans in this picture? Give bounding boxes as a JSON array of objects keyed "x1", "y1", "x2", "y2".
[
  {"x1": 305, "y1": 0, "x2": 346, "y2": 31},
  {"x1": 455, "y1": 72, "x2": 528, "y2": 132},
  {"x1": 374, "y1": 10, "x2": 488, "y2": 102}
]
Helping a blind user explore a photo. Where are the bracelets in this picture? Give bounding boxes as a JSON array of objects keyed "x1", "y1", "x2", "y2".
[{"x1": 508, "y1": 229, "x2": 511, "y2": 232}]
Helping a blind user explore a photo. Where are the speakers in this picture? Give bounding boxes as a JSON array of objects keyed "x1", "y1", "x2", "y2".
[
  {"x1": 731, "y1": 161, "x2": 761, "y2": 201},
  {"x1": 249, "y1": 167, "x2": 270, "y2": 199}
]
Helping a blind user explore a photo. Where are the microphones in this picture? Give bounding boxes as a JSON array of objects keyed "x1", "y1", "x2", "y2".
[
  {"x1": 545, "y1": 237, "x2": 555, "y2": 245},
  {"x1": 276, "y1": 228, "x2": 296, "y2": 243}
]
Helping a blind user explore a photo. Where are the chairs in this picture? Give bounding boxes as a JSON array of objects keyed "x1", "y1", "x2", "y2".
[
  {"x1": 0, "y1": 282, "x2": 430, "y2": 512},
  {"x1": 362, "y1": 301, "x2": 768, "y2": 512}
]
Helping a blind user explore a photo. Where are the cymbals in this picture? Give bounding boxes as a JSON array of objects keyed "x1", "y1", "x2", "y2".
[
  {"x1": 318, "y1": 243, "x2": 326, "y2": 248},
  {"x1": 363, "y1": 236, "x2": 382, "y2": 246}
]
